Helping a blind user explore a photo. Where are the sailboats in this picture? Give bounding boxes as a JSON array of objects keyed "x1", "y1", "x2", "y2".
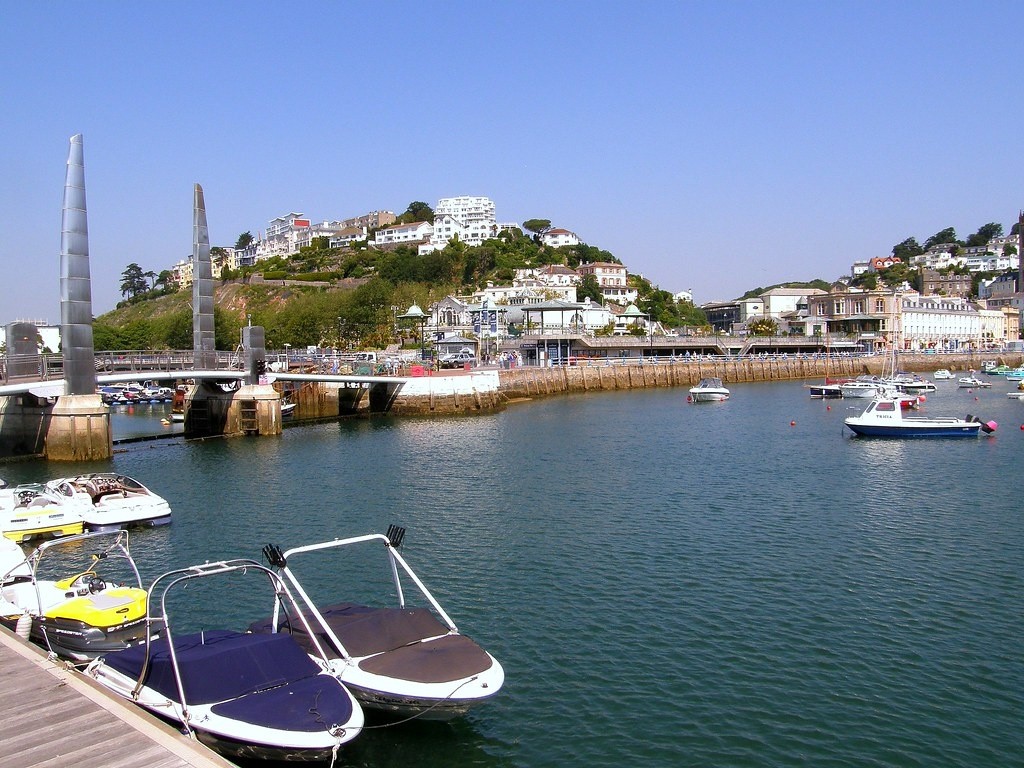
[{"x1": 803, "y1": 291, "x2": 937, "y2": 409}]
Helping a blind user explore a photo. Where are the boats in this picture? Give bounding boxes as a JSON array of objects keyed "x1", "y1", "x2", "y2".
[
  {"x1": 99, "y1": 382, "x2": 177, "y2": 405},
  {"x1": 0, "y1": 469, "x2": 506, "y2": 765},
  {"x1": 957, "y1": 370, "x2": 994, "y2": 388},
  {"x1": 279, "y1": 402, "x2": 298, "y2": 416},
  {"x1": 1006, "y1": 391, "x2": 1024, "y2": 400},
  {"x1": 980, "y1": 359, "x2": 1024, "y2": 388},
  {"x1": 843, "y1": 393, "x2": 999, "y2": 435},
  {"x1": 932, "y1": 369, "x2": 956, "y2": 380},
  {"x1": 686, "y1": 377, "x2": 730, "y2": 403}
]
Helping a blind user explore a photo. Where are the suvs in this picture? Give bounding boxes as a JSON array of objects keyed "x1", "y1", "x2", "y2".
[{"x1": 440, "y1": 354, "x2": 478, "y2": 369}]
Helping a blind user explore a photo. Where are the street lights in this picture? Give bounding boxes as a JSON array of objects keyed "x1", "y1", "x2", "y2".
[
  {"x1": 428, "y1": 303, "x2": 441, "y2": 373},
  {"x1": 284, "y1": 343, "x2": 291, "y2": 372}
]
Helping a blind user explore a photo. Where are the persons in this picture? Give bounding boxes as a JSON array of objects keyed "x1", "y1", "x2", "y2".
[
  {"x1": 499, "y1": 351, "x2": 517, "y2": 368},
  {"x1": 386, "y1": 357, "x2": 399, "y2": 375},
  {"x1": 686, "y1": 350, "x2": 696, "y2": 363},
  {"x1": 323, "y1": 357, "x2": 339, "y2": 374}
]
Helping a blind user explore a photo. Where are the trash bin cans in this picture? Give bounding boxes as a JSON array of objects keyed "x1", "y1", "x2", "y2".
[
  {"x1": 505, "y1": 361, "x2": 509, "y2": 368},
  {"x1": 511, "y1": 361, "x2": 514, "y2": 368}
]
